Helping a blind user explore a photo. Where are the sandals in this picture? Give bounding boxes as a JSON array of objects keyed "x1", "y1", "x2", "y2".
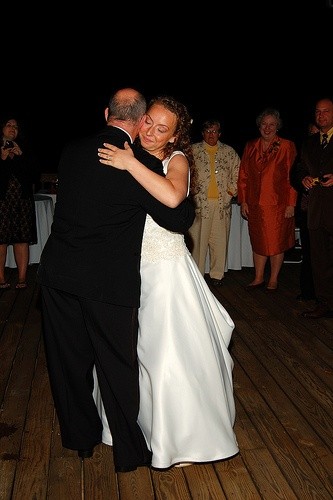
[
  {"x1": 0, "y1": 281, "x2": 11, "y2": 289},
  {"x1": 14, "y1": 282, "x2": 29, "y2": 289}
]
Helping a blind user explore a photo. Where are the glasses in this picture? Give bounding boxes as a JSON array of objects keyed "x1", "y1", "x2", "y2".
[{"x1": 206, "y1": 130, "x2": 216, "y2": 133}]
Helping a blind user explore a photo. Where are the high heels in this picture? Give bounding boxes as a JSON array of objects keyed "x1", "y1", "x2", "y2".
[
  {"x1": 263, "y1": 283, "x2": 278, "y2": 294},
  {"x1": 244, "y1": 280, "x2": 266, "y2": 290}
]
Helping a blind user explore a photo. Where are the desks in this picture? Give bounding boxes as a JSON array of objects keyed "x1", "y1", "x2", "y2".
[
  {"x1": 204, "y1": 199, "x2": 254, "y2": 274},
  {"x1": 5, "y1": 193, "x2": 56, "y2": 269}
]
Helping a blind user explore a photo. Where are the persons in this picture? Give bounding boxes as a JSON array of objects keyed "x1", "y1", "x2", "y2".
[
  {"x1": 35, "y1": 87, "x2": 198, "y2": 472},
  {"x1": 91, "y1": 95, "x2": 239, "y2": 470},
  {"x1": 0, "y1": 98, "x2": 333, "y2": 319}
]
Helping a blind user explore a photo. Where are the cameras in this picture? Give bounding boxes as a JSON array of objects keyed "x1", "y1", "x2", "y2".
[{"x1": 3, "y1": 140, "x2": 14, "y2": 150}]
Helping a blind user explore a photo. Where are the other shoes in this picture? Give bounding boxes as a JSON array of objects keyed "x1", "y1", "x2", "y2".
[
  {"x1": 296, "y1": 294, "x2": 315, "y2": 301},
  {"x1": 212, "y1": 278, "x2": 224, "y2": 288},
  {"x1": 301, "y1": 307, "x2": 333, "y2": 319}
]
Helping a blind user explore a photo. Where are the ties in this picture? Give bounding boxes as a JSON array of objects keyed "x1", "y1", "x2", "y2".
[{"x1": 322, "y1": 134, "x2": 328, "y2": 150}]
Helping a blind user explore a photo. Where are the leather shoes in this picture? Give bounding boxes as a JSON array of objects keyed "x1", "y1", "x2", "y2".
[
  {"x1": 114, "y1": 451, "x2": 152, "y2": 473},
  {"x1": 77, "y1": 449, "x2": 94, "y2": 458}
]
